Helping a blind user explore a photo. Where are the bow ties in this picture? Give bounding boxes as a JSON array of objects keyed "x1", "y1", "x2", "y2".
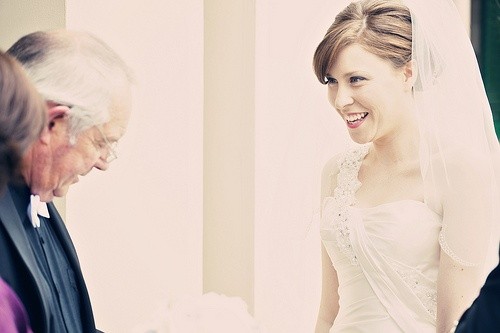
[{"x1": 26, "y1": 195, "x2": 50, "y2": 227}]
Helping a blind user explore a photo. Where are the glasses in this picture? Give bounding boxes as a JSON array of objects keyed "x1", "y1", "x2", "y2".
[{"x1": 95, "y1": 124, "x2": 119, "y2": 163}]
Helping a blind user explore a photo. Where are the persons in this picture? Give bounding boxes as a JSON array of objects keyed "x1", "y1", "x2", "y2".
[
  {"x1": 313, "y1": 1, "x2": 495, "y2": 333},
  {"x1": 1, "y1": 52, "x2": 46, "y2": 333},
  {"x1": 0, "y1": 28, "x2": 132, "y2": 333}
]
